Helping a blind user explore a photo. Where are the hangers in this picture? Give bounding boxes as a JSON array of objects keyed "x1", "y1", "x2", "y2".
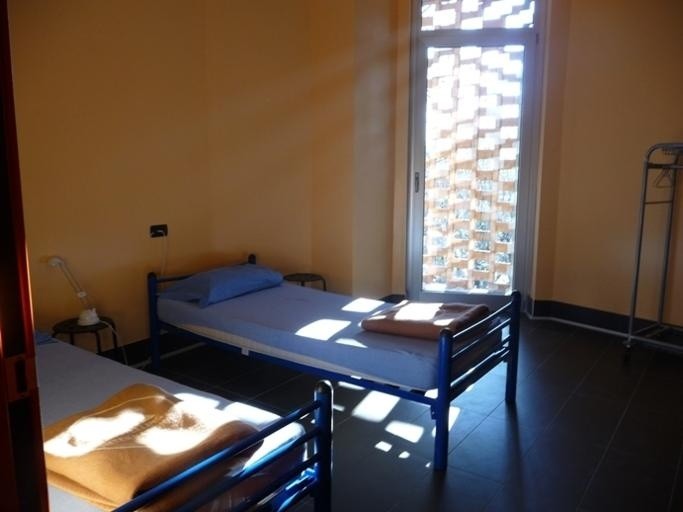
[{"x1": 647, "y1": 148, "x2": 677, "y2": 190}]
